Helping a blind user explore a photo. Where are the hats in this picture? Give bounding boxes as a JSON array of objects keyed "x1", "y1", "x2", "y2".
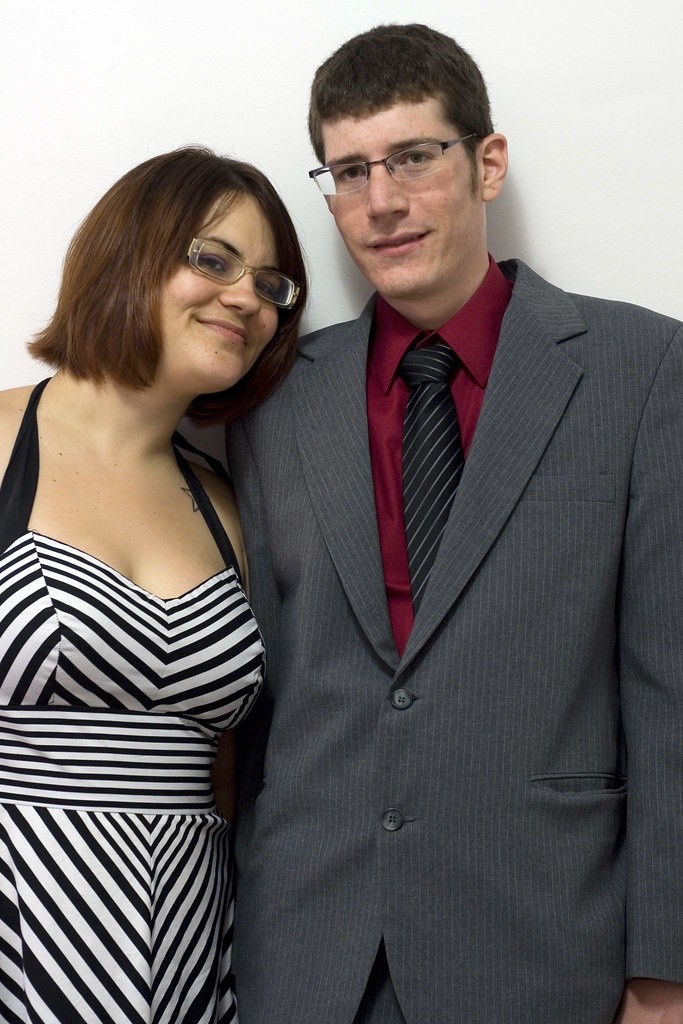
[{"x1": 396, "y1": 341, "x2": 467, "y2": 618}]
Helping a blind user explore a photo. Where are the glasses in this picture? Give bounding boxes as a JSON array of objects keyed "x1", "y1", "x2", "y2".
[
  {"x1": 309, "y1": 132, "x2": 480, "y2": 196},
  {"x1": 183, "y1": 238, "x2": 300, "y2": 310}
]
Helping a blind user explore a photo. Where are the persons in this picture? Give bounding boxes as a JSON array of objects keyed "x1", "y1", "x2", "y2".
[
  {"x1": 0, "y1": 144, "x2": 308, "y2": 1024},
  {"x1": 216, "y1": 22, "x2": 683, "y2": 1024}
]
